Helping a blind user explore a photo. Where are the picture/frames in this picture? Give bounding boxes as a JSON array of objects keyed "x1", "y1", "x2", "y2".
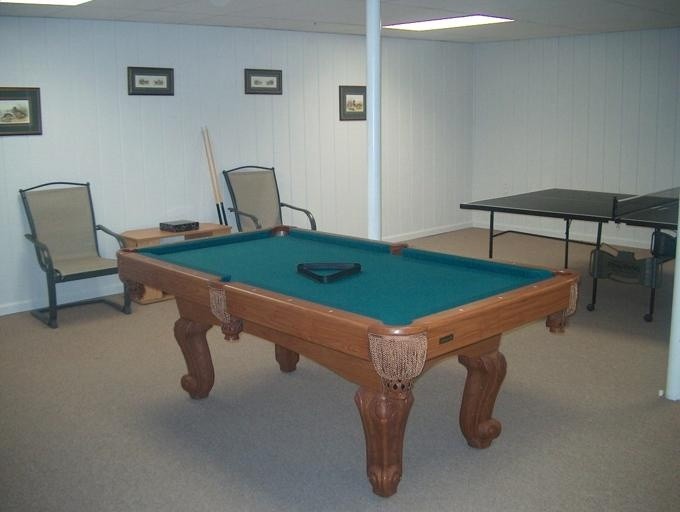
[
  {"x1": 339, "y1": 86, "x2": 367, "y2": 120},
  {"x1": 127, "y1": 67, "x2": 174, "y2": 95},
  {"x1": 0, "y1": 86, "x2": 42, "y2": 136},
  {"x1": 244, "y1": 69, "x2": 283, "y2": 95}
]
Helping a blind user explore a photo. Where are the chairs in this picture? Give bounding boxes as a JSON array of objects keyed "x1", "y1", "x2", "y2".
[
  {"x1": 223, "y1": 166, "x2": 316, "y2": 232},
  {"x1": 19, "y1": 182, "x2": 132, "y2": 329}
]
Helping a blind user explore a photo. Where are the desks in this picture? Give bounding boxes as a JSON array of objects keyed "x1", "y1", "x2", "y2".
[
  {"x1": 120, "y1": 223, "x2": 233, "y2": 305},
  {"x1": 460, "y1": 188, "x2": 679, "y2": 322},
  {"x1": 115, "y1": 225, "x2": 581, "y2": 498}
]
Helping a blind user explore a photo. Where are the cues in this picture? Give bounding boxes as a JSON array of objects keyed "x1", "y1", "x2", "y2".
[{"x1": 199, "y1": 123, "x2": 228, "y2": 225}]
